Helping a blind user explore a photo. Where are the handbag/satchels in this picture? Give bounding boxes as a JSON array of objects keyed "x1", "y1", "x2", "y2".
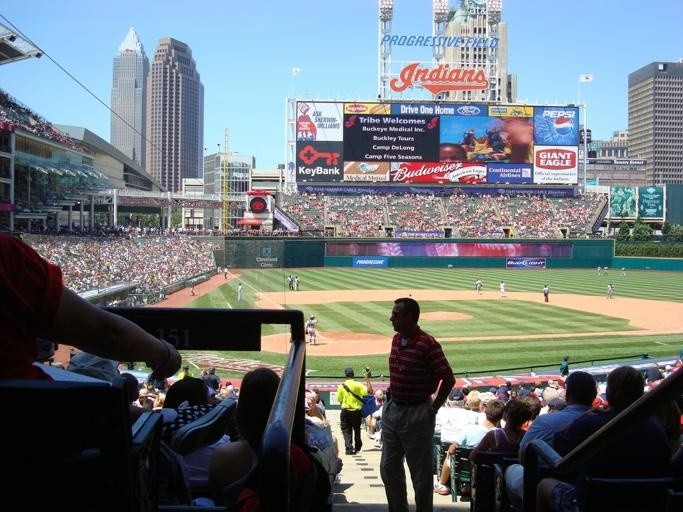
[{"x1": 361, "y1": 396, "x2": 376, "y2": 416}]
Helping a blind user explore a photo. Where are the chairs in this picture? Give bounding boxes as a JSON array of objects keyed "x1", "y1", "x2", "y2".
[
  {"x1": 426, "y1": 434, "x2": 680, "y2": 510},
  {"x1": 5, "y1": 380, "x2": 168, "y2": 510}
]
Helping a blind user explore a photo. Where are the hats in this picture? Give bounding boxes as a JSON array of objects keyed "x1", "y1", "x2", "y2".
[
  {"x1": 68, "y1": 349, "x2": 120, "y2": 383},
  {"x1": 449, "y1": 390, "x2": 463, "y2": 400},
  {"x1": 345, "y1": 367, "x2": 354, "y2": 376}
]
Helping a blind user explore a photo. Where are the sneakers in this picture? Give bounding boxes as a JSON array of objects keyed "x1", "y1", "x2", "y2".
[
  {"x1": 346, "y1": 448, "x2": 355, "y2": 454},
  {"x1": 369, "y1": 432, "x2": 381, "y2": 439},
  {"x1": 356, "y1": 441, "x2": 362, "y2": 451},
  {"x1": 433, "y1": 482, "x2": 449, "y2": 495}
]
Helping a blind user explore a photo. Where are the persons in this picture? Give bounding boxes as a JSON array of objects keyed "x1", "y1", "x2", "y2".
[
  {"x1": 440, "y1": 116, "x2": 534, "y2": 164},
  {"x1": 475, "y1": 278, "x2": 483, "y2": 295},
  {"x1": 597, "y1": 265, "x2": 627, "y2": 277},
  {"x1": 336, "y1": 350, "x2": 683, "y2": 512},
  {"x1": 1, "y1": 88, "x2": 222, "y2": 308},
  {"x1": 380, "y1": 297, "x2": 456, "y2": 512},
  {"x1": 1, "y1": 226, "x2": 342, "y2": 512},
  {"x1": 408, "y1": 294, "x2": 415, "y2": 300},
  {"x1": 500, "y1": 280, "x2": 505, "y2": 298},
  {"x1": 543, "y1": 284, "x2": 549, "y2": 303},
  {"x1": 223, "y1": 190, "x2": 608, "y2": 239},
  {"x1": 607, "y1": 283, "x2": 614, "y2": 299}
]
